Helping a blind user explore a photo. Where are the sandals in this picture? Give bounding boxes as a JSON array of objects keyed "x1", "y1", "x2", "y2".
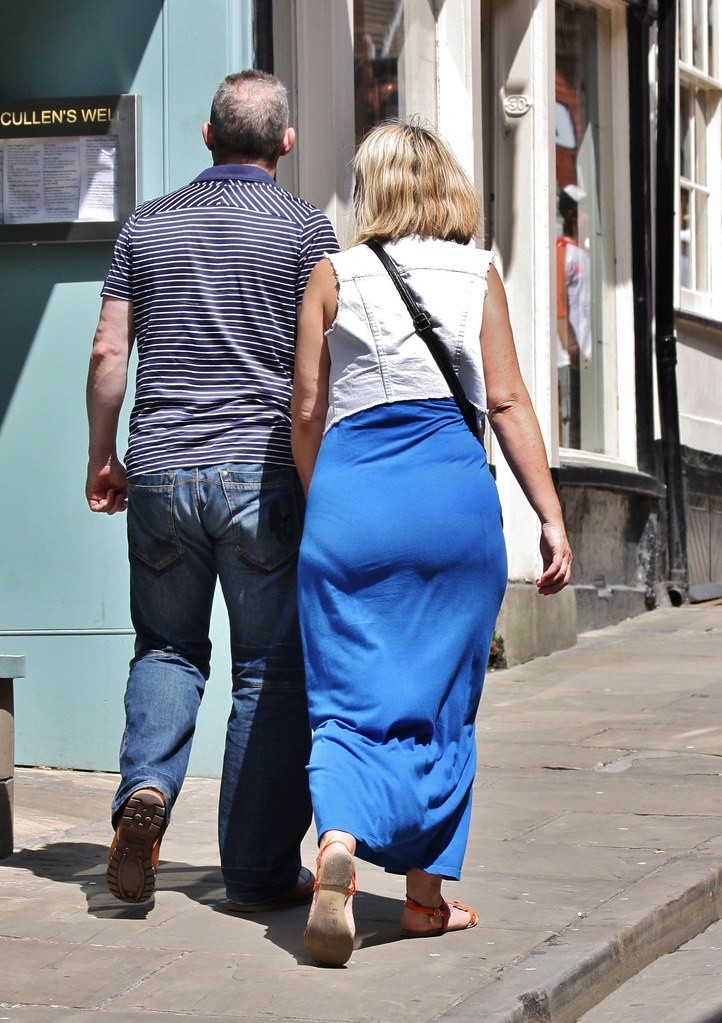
[
  {"x1": 400, "y1": 894, "x2": 479, "y2": 937},
  {"x1": 305, "y1": 841, "x2": 356, "y2": 965}
]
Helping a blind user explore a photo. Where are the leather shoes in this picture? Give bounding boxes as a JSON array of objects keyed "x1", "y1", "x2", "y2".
[
  {"x1": 227, "y1": 867, "x2": 315, "y2": 913},
  {"x1": 106, "y1": 789, "x2": 165, "y2": 903}
]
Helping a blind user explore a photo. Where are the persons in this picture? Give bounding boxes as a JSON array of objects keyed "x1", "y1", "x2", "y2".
[
  {"x1": 294, "y1": 124, "x2": 573, "y2": 964},
  {"x1": 87, "y1": 69, "x2": 339, "y2": 909},
  {"x1": 557, "y1": 198, "x2": 580, "y2": 450}
]
